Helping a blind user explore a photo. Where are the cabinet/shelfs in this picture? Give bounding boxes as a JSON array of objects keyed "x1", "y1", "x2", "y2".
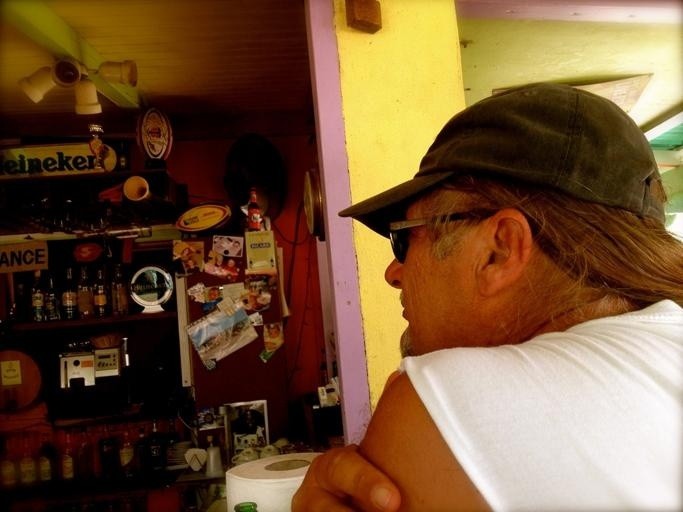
[{"x1": 0, "y1": 271, "x2": 198, "y2": 430}]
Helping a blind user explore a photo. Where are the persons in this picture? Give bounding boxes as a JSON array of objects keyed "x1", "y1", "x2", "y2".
[
  {"x1": 214, "y1": 236, "x2": 242, "y2": 275},
  {"x1": 291, "y1": 83, "x2": 683, "y2": 512},
  {"x1": 267, "y1": 325, "x2": 282, "y2": 348},
  {"x1": 176, "y1": 246, "x2": 202, "y2": 272},
  {"x1": 205, "y1": 287, "x2": 223, "y2": 308}
]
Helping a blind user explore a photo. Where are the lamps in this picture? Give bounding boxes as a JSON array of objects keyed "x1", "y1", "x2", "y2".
[{"x1": 20, "y1": 55, "x2": 138, "y2": 116}]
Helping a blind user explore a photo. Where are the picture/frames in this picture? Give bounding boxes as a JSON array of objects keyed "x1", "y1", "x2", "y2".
[{"x1": 223, "y1": 399, "x2": 270, "y2": 466}]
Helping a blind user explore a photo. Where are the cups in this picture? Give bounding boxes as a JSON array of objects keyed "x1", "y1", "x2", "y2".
[
  {"x1": 231, "y1": 446, "x2": 280, "y2": 465},
  {"x1": 205, "y1": 446, "x2": 225, "y2": 478}
]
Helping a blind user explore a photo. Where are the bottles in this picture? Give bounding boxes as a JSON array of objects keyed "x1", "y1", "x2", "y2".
[
  {"x1": 0, "y1": 416, "x2": 182, "y2": 491},
  {"x1": 247, "y1": 187, "x2": 261, "y2": 232},
  {"x1": 31, "y1": 262, "x2": 128, "y2": 323}
]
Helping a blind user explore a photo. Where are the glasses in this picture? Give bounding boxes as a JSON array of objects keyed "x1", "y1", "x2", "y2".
[{"x1": 389, "y1": 207, "x2": 497, "y2": 263}]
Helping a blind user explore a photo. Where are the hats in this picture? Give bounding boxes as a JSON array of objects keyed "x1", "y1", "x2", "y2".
[{"x1": 338, "y1": 83, "x2": 667, "y2": 236}]
